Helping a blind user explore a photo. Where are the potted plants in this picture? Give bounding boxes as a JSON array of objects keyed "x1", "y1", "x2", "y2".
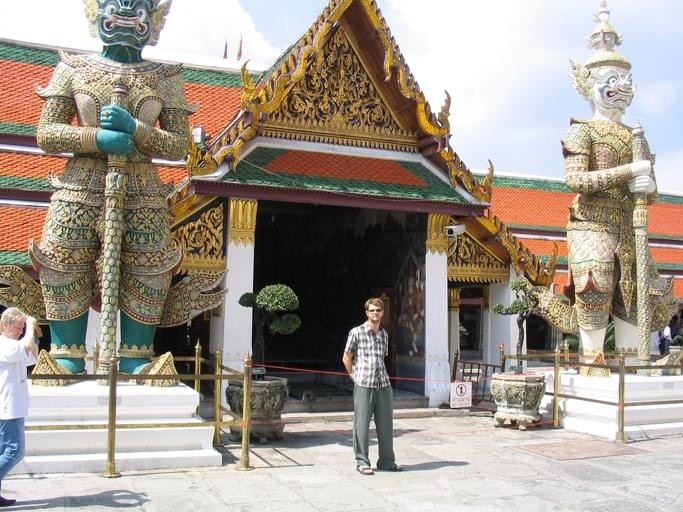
[
  {"x1": 493, "y1": 277, "x2": 545, "y2": 429},
  {"x1": 224, "y1": 283, "x2": 301, "y2": 441}
]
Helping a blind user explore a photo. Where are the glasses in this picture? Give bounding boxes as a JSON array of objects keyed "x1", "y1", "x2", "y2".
[{"x1": 368, "y1": 309, "x2": 382, "y2": 312}]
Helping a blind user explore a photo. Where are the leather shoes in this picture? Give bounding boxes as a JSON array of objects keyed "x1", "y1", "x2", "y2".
[{"x1": 0, "y1": 496, "x2": 16, "y2": 507}]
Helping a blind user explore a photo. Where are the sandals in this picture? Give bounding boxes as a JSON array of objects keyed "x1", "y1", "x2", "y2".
[
  {"x1": 356, "y1": 465, "x2": 374, "y2": 475},
  {"x1": 377, "y1": 462, "x2": 403, "y2": 471}
]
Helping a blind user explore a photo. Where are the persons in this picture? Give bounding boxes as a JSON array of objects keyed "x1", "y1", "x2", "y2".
[
  {"x1": 657, "y1": 328, "x2": 666, "y2": 358},
  {"x1": 0, "y1": 304, "x2": 42, "y2": 504},
  {"x1": 661, "y1": 323, "x2": 671, "y2": 356},
  {"x1": 341, "y1": 296, "x2": 403, "y2": 477}
]
